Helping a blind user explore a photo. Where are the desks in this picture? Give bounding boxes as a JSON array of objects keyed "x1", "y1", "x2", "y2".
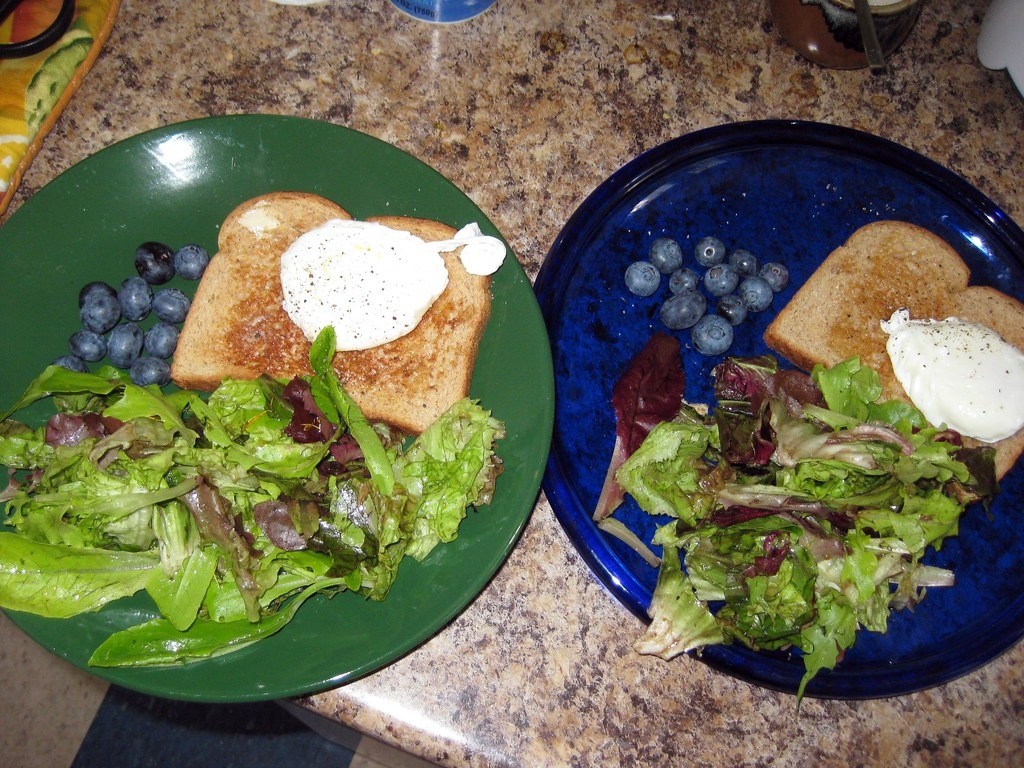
[{"x1": 0, "y1": 0, "x2": 1024, "y2": 768}]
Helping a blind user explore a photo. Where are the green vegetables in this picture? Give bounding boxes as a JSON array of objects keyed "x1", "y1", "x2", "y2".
[
  {"x1": 617, "y1": 358, "x2": 982, "y2": 722},
  {"x1": 1, "y1": 326, "x2": 506, "y2": 667}
]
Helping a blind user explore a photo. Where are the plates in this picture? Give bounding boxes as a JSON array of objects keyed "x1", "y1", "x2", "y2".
[
  {"x1": 0, "y1": 112, "x2": 553, "y2": 700},
  {"x1": 535, "y1": 122, "x2": 1024, "y2": 697}
]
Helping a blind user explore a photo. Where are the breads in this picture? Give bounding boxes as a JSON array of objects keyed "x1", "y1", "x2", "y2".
[
  {"x1": 171, "y1": 191, "x2": 492, "y2": 435},
  {"x1": 767, "y1": 220, "x2": 1024, "y2": 484}
]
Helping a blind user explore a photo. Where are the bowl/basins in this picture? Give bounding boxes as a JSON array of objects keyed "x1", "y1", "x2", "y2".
[{"x1": 772, "y1": 0, "x2": 921, "y2": 70}]
